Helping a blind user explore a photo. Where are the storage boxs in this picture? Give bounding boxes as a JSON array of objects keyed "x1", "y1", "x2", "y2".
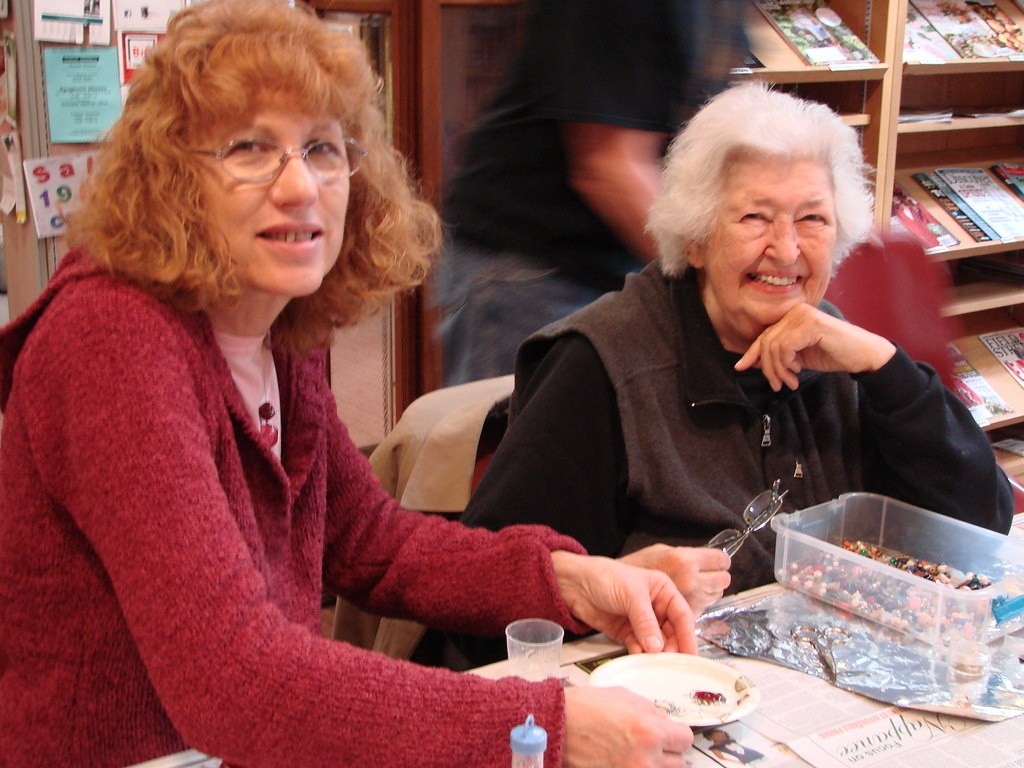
[{"x1": 771, "y1": 491, "x2": 1024, "y2": 645}]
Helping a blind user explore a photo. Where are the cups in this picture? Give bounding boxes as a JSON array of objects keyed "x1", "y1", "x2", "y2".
[{"x1": 505, "y1": 618, "x2": 564, "y2": 686}]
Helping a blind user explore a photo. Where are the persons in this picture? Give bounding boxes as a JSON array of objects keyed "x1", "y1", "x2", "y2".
[
  {"x1": 0, "y1": 0, "x2": 699, "y2": 768},
  {"x1": 444, "y1": 79, "x2": 1014, "y2": 674},
  {"x1": 433, "y1": 0, "x2": 754, "y2": 388}
]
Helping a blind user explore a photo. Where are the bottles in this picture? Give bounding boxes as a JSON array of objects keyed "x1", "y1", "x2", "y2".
[{"x1": 508, "y1": 716, "x2": 549, "y2": 768}]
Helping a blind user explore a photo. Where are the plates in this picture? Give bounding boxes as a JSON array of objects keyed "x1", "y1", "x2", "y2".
[{"x1": 589, "y1": 651, "x2": 752, "y2": 734}]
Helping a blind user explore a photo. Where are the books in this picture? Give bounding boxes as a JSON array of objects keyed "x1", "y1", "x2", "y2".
[
  {"x1": 898, "y1": 104, "x2": 1024, "y2": 124},
  {"x1": 939, "y1": 327, "x2": 1024, "y2": 469},
  {"x1": 900, "y1": 0, "x2": 1022, "y2": 64},
  {"x1": 754, "y1": 0, "x2": 881, "y2": 65},
  {"x1": 889, "y1": 161, "x2": 1024, "y2": 252}
]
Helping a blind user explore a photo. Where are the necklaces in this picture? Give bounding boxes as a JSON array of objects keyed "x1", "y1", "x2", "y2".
[{"x1": 223, "y1": 346, "x2": 281, "y2": 450}]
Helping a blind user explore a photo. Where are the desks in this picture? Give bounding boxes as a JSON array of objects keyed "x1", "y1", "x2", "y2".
[{"x1": 117, "y1": 581, "x2": 1024, "y2": 768}]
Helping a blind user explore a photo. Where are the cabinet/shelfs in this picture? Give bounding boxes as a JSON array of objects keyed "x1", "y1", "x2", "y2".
[{"x1": 725, "y1": 0, "x2": 1024, "y2": 476}]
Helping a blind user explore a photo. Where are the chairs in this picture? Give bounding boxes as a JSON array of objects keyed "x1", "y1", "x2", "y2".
[{"x1": 328, "y1": 374, "x2": 516, "y2": 672}]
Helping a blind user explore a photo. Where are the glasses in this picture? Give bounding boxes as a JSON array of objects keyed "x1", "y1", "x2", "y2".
[
  {"x1": 704, "y1": 479, "x2": 790, "y2": 557},
  {"x1": 190, "y1": 137, "x2": 368, "y2": 183}
]
